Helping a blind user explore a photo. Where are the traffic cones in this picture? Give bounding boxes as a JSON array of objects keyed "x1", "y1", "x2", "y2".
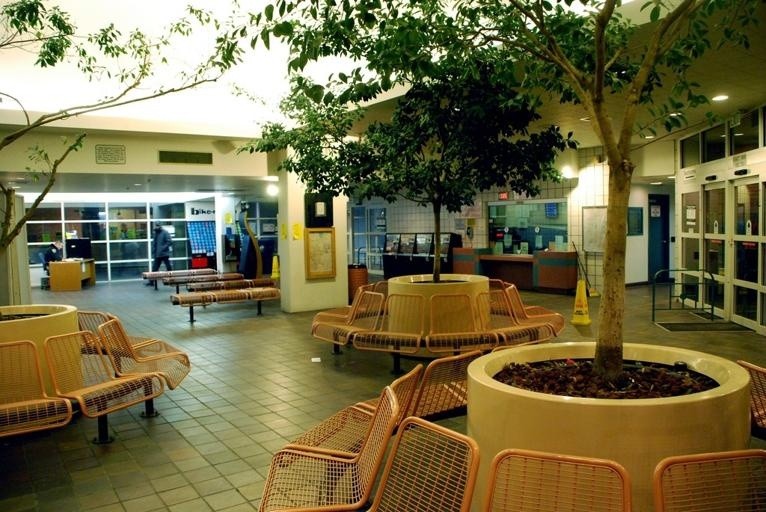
[
  {"x1": 269, "y1": 254, "x2": 280, "y2": 279},
  {"x1": 568, "y1": 279, "x2": 594, "y2": 326}
]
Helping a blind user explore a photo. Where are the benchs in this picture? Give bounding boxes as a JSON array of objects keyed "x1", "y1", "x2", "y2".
[{"x1": 142, "y1": 268, "x2": 282, "y2": 322}]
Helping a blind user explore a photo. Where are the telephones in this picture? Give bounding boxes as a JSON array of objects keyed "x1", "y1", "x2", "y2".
[{"x1": 466, "y1": 227, "x2": 470, "y2": 235}]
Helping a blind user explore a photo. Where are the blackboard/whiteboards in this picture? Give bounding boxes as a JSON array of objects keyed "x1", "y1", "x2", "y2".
[{"x1": 581, "y1": 206, "x2": 608, "y2": 253}]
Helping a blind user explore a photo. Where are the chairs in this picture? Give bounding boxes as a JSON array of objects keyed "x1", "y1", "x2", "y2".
[
  {"x1": 38, "y1": 252, "x2": 49, "y2": 276},
  {"x1": 1, "y1": 309, "x2": 191, "y2": 446}
]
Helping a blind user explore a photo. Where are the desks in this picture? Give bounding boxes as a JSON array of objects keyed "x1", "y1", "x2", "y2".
[{"x1": 48, "y1": 258, "x2": 96, "y2": 291}]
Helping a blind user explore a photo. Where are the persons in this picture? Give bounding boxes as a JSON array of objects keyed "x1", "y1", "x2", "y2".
[
  {"x1": 117, "y1": 223, "x2": 142, "y2": 276},
  {"x1": 144, "y1": 222, "x2": 173, "y2": 285},
  {"x1": 44, "y1": 240, "x2": 64, "y2": 269}
]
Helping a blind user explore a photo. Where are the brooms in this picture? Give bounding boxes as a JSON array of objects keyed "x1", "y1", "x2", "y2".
[{"x1": 572, "y1": 240, "x2": 600, "y2": 297}]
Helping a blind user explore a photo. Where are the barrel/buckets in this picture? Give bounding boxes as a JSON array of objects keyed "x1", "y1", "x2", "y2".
[{"x1": 191, "y1": 252, "x2": 208, "y2": 269}]
